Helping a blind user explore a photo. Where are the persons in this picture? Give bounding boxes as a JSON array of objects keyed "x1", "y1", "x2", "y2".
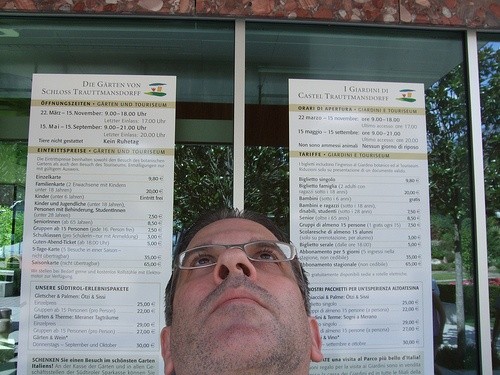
[
  {"x1": 404, "y1": 282, "x2": 449, "y2": 375},
  {"x1": 157, "y1": 200, "x2": 324, "y2": 374},
  {"x1": 403, "y1": 268, "x2": 445, "y2": 352}
]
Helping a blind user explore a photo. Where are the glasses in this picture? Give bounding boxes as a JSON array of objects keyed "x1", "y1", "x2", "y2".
[{"x1": 168, "y1": 240, "x2": 306, "y2": 299}]
